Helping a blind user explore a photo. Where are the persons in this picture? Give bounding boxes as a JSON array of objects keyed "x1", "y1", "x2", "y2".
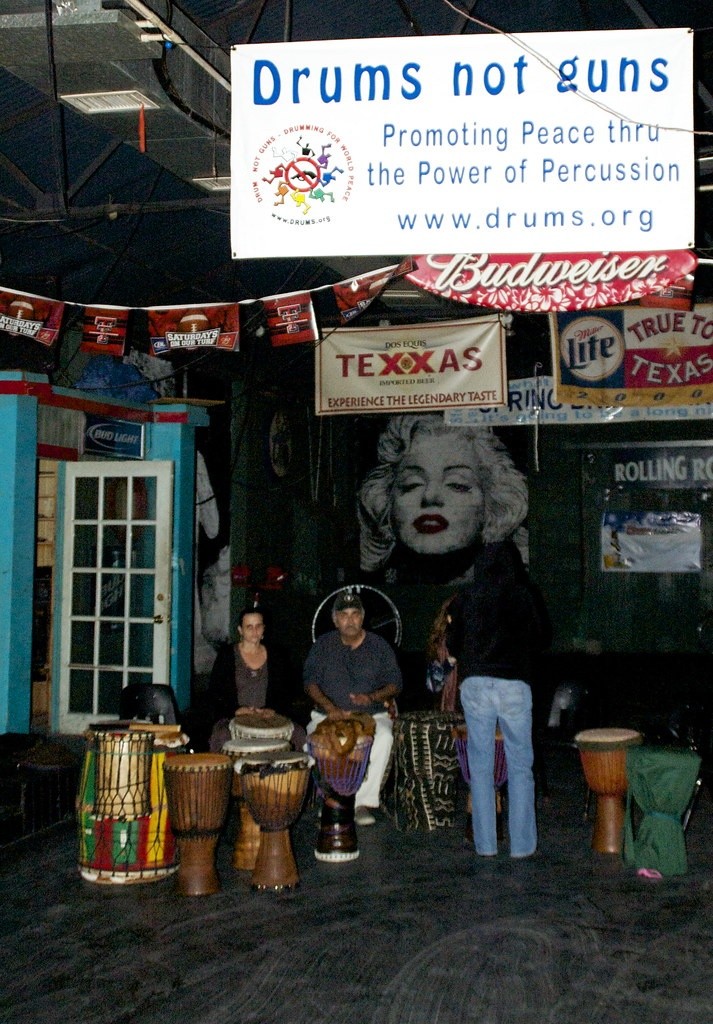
[
  {"x1": 359, "y1": 416, "x2": 528, "y2": 583},
  {"x1": 447, "y1": 540, "x2": 555, "y2": 857},
  {"x1": 208, "y1": 607, "x2": 282, "y2": 718},
  {"x1": 304, "y1": 594, "x2": 403, "y2": 825}
]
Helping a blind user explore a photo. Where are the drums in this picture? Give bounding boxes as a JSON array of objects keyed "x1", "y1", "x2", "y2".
[
  {"x1": 391, "y1": 709, "x2": 468, "y2": 838},
  {"x1": 304, "y1": 713, "x2": 377, "y2": 863},
  {"x1": 234, "y1": 753, "x2": 317, "y2": 893},
  {"x1": 453, "y1": 722, "x2": 508, "y2": 842},
  {"x1": 574, "y1": 727, "x2": 646, "y2": 855},
  {"x1": 221, "y1": 741, "x2": 292, "y2": 872},
  {"x1": 71, "y1": 722, "x2": 196, "y2": 888},
  {"x1": 228, "y1": 712, "x2": 296, "y2": 742},
  {"x1": 625, "y1": 745, "x2": 703, "y2": 876},
  {"x1": 160, "y1": 753, "x2": 233, "y2": 898}
]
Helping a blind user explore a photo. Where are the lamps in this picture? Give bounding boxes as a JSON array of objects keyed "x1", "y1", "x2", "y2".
[{"x1": 103, "y1": 194, "x2": 117, "y2": 221}]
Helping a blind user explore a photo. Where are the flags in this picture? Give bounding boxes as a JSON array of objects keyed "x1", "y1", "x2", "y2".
[{"x1": 0, "y1": 256, "x2": 414, "y2": 354}]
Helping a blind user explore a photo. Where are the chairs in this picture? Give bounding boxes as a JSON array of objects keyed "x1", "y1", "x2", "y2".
[{"x1": 121, "y1": 683, "x2": 185, "y2": 731}]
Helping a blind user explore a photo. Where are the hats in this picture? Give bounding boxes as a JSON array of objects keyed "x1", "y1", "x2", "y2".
[{"x1": 334, "y1": 593, "x2": 363, "y2": 612}]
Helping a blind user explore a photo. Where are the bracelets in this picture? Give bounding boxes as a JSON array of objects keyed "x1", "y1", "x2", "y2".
[{"x1": 369, "y1": 695, "x2": 373, "y2": 703}]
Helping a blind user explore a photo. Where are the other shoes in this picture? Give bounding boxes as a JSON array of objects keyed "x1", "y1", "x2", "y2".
[{"x1": 354, "y1": 805, "x2": 376, "y2": 826}]
